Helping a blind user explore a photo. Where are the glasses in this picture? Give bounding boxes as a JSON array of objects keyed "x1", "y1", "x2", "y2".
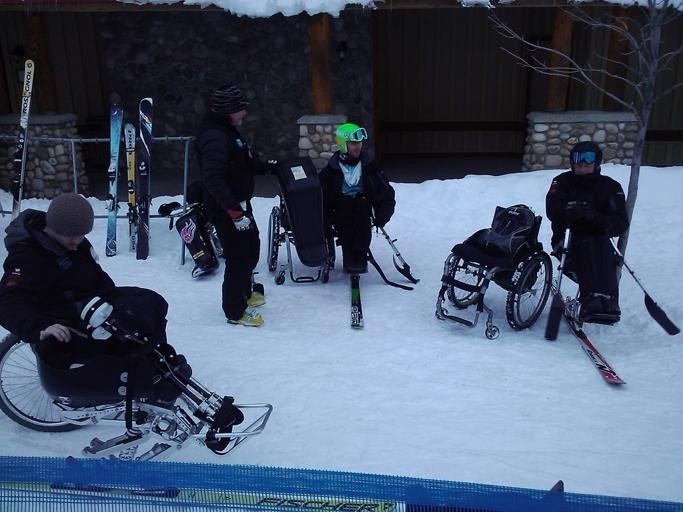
[
  {"x1": 570, "y1": 150, "x2": 598, "y2": 164},
  {"x1": 344, "y1": 127, "x2": 368, "y2": 142}
]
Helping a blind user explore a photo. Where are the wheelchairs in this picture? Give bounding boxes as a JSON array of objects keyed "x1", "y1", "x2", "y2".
[
  {"x1": 0, "y1": 319, "x2": 276, "y2": 456},
  {"x1": 261, "y1": 151, "x2": 340, "y2": 284},
  {"x1": 435, "y1": 204, "x2": 554, "y2": 340}
]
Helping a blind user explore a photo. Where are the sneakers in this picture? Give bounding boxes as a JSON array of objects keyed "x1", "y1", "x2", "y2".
[
  {"x1": 246, "y1": 292, "x2": 267, "y2": 308},
  {"x1": 227, "y1": 308, "x2": 264, "y2": 327}
]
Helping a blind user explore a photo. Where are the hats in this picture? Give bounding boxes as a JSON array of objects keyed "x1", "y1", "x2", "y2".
[
  {"x1": 46, "y1": 193, "x2": 94, "y2": 236},
  {"x1": 212, "y1": 83, "x2": 251, "y2": 114}
]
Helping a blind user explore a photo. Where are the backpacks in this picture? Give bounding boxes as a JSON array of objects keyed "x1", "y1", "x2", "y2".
[{"x1": 477, "y1": 204, "x2": 536, "y2": 258}]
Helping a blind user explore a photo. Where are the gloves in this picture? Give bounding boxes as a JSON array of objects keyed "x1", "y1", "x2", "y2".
[{"x1": 232, "y1": 215, "x2": 251, "y2": 231}]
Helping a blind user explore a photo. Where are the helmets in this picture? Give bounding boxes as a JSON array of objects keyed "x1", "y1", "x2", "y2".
[
  {"x1": 570, "y1": 141, "x2": 602, "y2": 170},
  {"x1": 336, "y1": 123, "x2": 360, "y2": 154}
]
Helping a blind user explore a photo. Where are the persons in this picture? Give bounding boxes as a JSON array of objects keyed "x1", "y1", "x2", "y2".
[
  {"x1": 543, "y1": 142, "x2": 630, "y2": 325},
  {"x1": 317, "y1": 124, "x2": 396, "y2": 254},
  {"x1": 0, "y1": 193, "x2": 192, "y2": 406},
  {"x1": 196, "y1": 86, "x2": 279, "y2": 328}
]
